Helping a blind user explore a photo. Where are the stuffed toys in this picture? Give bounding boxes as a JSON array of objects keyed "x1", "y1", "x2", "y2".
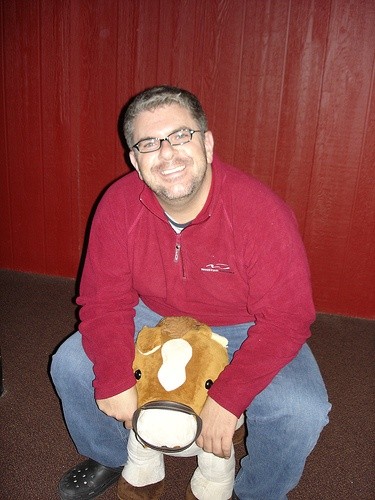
[{"x1": 113, "y1": 315, "x2": 246, "y2": 500}]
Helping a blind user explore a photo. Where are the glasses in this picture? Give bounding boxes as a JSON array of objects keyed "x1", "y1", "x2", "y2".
[{"x1": 132, "y1": 128, "x2": 205, "y2": 153}]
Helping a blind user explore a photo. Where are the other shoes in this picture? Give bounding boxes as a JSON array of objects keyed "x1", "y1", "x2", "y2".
[{"x1": 59, "y1": 458, "x2": 124, "y2": 500}]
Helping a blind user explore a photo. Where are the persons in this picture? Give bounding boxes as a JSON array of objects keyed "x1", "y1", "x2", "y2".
[{"x1": 47, "y1": 85, "x2": 334, "y2": 500}]
